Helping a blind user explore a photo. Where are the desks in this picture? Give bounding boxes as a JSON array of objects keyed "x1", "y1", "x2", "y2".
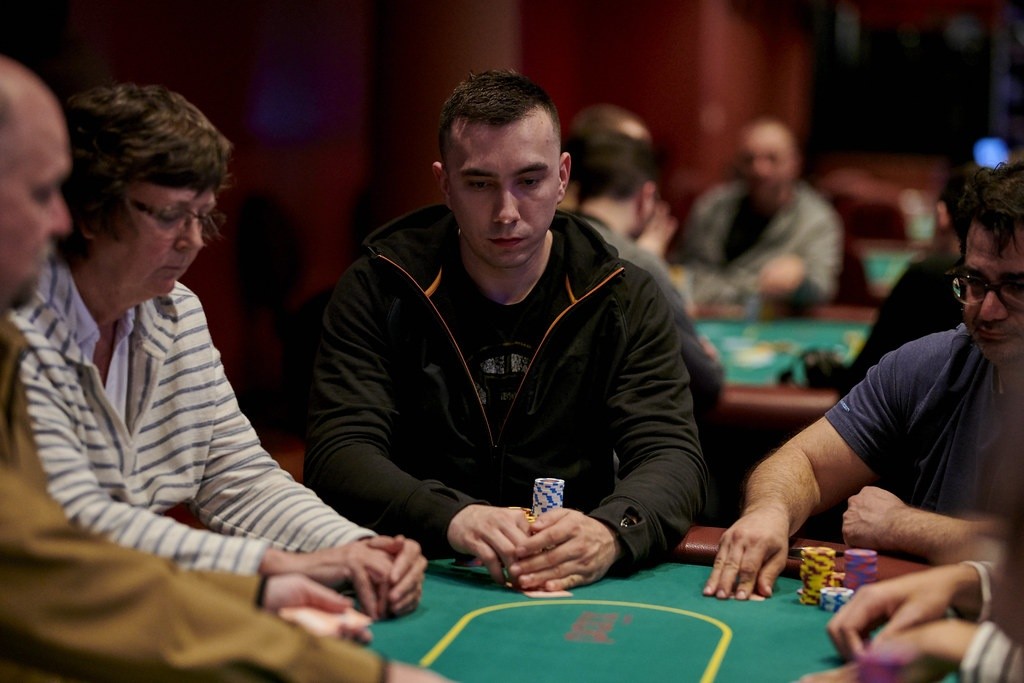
[
  {"x1": 835, "y1": 238, "x2": 937, "y2": 294},
  {"x1": 337, "y1": 523, "x2": 965, "y2": 683},
  {"x1": 670, "y1": 298, "x2": 908, "y2": 428}
]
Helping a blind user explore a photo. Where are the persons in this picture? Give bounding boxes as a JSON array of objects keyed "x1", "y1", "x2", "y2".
[
  {"x1": 0, "y1": 56, "x2": 450, "y2": 683},
  {"x1": 683, "y1": 117, "x2": 845, "y2": 309},
  {"x1": 558, "y1": 106, "x2": 725, "y2": 407},
  {"x1": 703, "y1": 159, "x2": 1024, "y2": 601},
  {"x1": 303, "y1": 70, "x2": 709, "y2": 592},
  {"x1": 793, "y1": 561, "x2": 1024, "y2": 683},
  {"x1": 9, "y1": 84, "x2": 427, "y2": 618},
  {"x1": 835, "y1": 164, "x2": 977, "y2": 408}
]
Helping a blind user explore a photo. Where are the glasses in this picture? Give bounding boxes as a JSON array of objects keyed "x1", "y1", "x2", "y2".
[
  {"x1": 124, "y1": 193, "x2": 226, "y2": 242},
  {"x1": 951, "y1": 267, "x2": 1024, "y2": 311}
]
